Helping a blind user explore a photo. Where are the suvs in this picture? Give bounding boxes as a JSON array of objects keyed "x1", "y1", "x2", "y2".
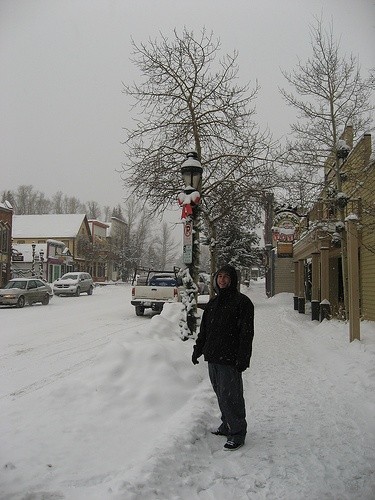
[{"x1": 54, "y1": 271, "x2": 94, "y2": 297}]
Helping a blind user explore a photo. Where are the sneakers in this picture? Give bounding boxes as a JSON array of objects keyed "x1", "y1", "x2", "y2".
[
  {"x1": 223, "y1": 438, "x2": 245, "y2": 451},
  {"x1": 212, "y1": 430, "x2": 228, "y2": 437}
]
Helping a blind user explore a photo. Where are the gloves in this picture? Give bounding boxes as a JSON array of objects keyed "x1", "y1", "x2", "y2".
[
  {"x1": 191, "y1": 349, "x2": 202, "y2": 365},
  {"x1": 236, "y1": 362, "x2": 249, "y2": 373}
]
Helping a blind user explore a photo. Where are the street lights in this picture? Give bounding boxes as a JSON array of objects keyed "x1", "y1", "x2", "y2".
[{"x1": 179, "y1": 152, "x2": 204, "y2": 340}]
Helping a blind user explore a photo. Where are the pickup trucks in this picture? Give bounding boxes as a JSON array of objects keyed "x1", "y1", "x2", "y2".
[{"x1": 130, "y1": 265, "x2": 184, "y2": 315}]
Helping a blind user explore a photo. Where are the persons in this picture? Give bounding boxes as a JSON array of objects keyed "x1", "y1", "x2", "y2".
[{"x1": 191, "y1": 265, "x2": 254, "y2": 451}]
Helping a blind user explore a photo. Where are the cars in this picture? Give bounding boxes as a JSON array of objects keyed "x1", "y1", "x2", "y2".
[{"x1": 0, "y1": 278, "x2": 51, "y2": 308}]
